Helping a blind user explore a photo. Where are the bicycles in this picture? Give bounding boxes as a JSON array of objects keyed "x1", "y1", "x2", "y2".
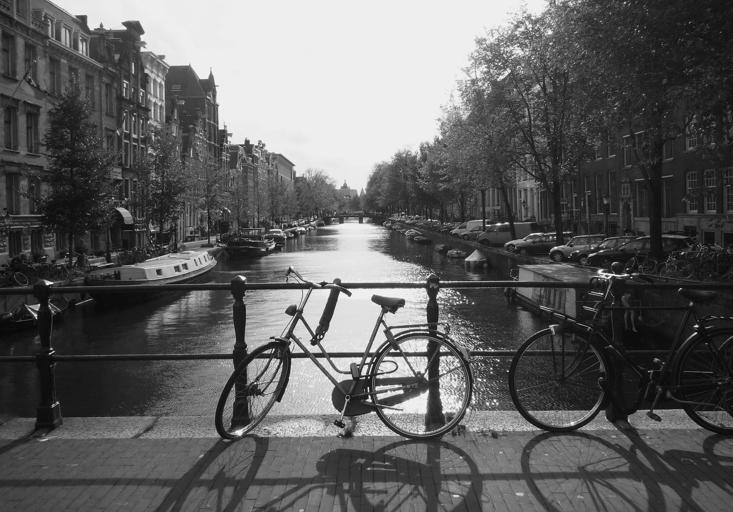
[
  {"x1": 509, "y1": 258, "x2": 732, "y2": 436},
  {"x1": 0, "y1": 252, "x2": 90, "y2": 288},
  {"x1": 214, "y1": 265, "x2": 474, "y2": 441}
]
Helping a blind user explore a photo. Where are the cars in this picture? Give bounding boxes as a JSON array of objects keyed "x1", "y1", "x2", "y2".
[{"x1": 503, "y1": 232, "x2": 695, "y2": 268}]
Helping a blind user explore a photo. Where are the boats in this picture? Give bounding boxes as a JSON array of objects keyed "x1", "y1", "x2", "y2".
[
  {"x1": 84, "y1": 248, "x2": 217, "y2": 301},
  {"x1": 227, "y1": 220, "x2": 325, "y2": 256},
  {"x1": 0, "y1": 293, "x2": 71, "y2": 327},
  {"x1": 382, "y1": 220, "x2": 427, "y2": 242},
  {"x1": 434, "y1": 243, "x2": 467, "y2": 258}
]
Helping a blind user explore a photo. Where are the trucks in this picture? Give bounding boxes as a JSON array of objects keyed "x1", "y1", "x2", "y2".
[{"x1": 450, "y1": 219, "x2": 536, "y2": 246}]
